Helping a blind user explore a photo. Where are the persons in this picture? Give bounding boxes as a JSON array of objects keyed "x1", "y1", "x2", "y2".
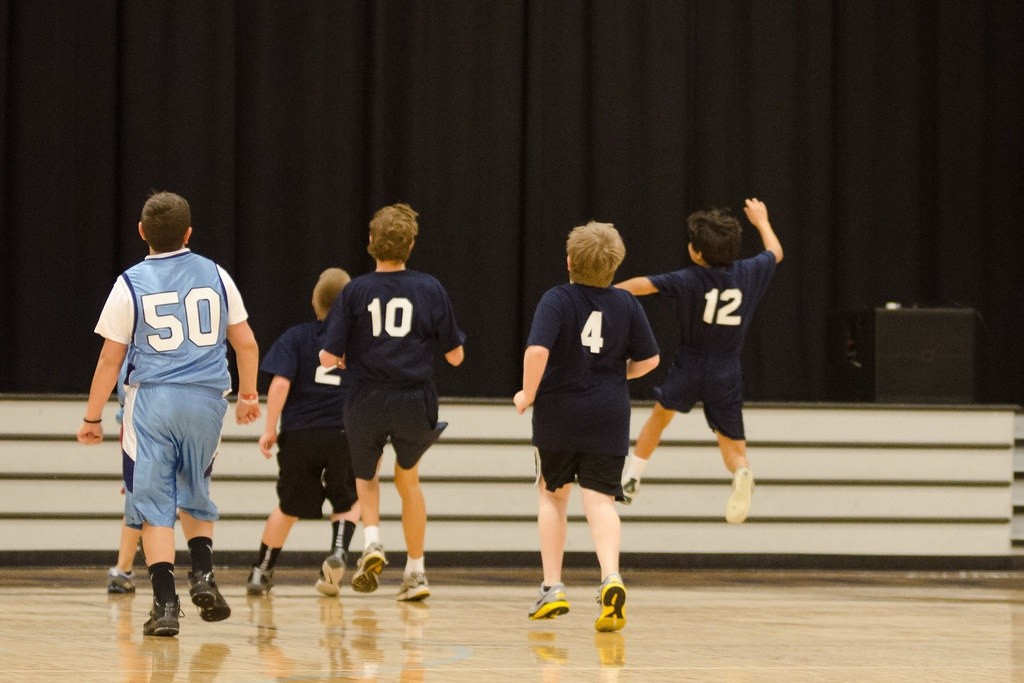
[
  {"x1": 107, "y1": 343, "x2": 145, "y2": 593},
  {"x1": 245, "y1": 266, "x2": 361, "y2": 596},
  {"x1": 78, "y1": 193, "x2": 259, "y2": 635},
  {"x1": 318, "y1": 205, "x2": 465, "y2": 600},
  {"x1": 514, "y1": 222, "x2": 660, "y2": 631},
  {"x1": 614, "y1": 198, "x2": 783, "y2": 525}
]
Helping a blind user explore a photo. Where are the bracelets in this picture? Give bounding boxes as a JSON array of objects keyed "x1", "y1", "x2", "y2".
[
  {"x1": 236, "y1": 392, "x2": 258, "y2": 399},
  {"x1": 84, "y1": 418, "x2": 101, "y2": 423},
  {"x1": 238, "y1": 397, "x2": 258, "y2": 404}
]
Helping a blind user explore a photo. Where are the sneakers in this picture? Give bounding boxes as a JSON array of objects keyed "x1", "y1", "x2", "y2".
[
  {"x1": 619, "y1": 473, "x2": 640, "y2": 506},
  {"x1": 529, "y1": 632, "x2": 567, "y2": 666},
  {"x1": 594, "y1": 631, "x2": 627, "y2": 668},
  {"x1": 246, "y1": 567, "x2": 274, "y2": 593},
  {"x1": 317, "y1": 599, "x2": 345, "y2": 648},
  {"x1": 528, "y1": 580, "x2": 570, "y2": 621},
  {"x1": 396, "y1": 569, "x2": 430, "y2": 601},
  {"x1": 725, "y1": 467, "x2": 754, "y2": 525},
  {"x1": 248, "y1": 597, "x2": 277, "y2": 644},
  {"x1": 594, "y1": 573, "x2": 627, "y2": 631},
  {"x1": 313, "y1": 547, "x2": 347, "y2": 597},
  {"x1": 187, "y1": 569, "x2": 231, "y2": 623},
  {"x1": 142, "y1": 593, "x2": 181, "y2": 635},
  {"x1": 352, "y1": 542, "x2": 388, "y2": 592},
  {"x1": 191, "y1": 643, "x2": 230, "y2": 676},
  {"x1": 107, "y1": 566, "x2": 136, "y2": 593},
  {"x1": 397, "y1": 600, "x2": 431, "y2": 623},
  {"x1": 152, "y1": 637, "x2": 179, "y2": 676}
]
